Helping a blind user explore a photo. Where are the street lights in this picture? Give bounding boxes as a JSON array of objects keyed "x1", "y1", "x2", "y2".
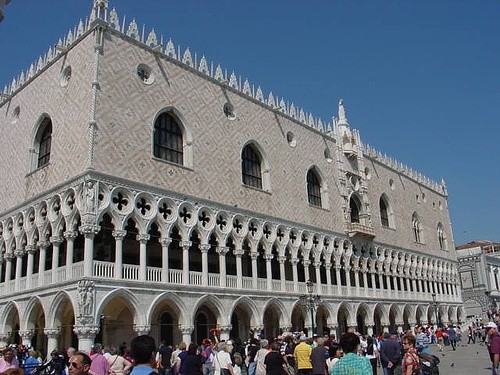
[
  {"x1": 299, "y1": 279, "x2": 322, "y2": 338},
  {"x1": 429, "y1": 293, "x2": 441, "y2": 325}
]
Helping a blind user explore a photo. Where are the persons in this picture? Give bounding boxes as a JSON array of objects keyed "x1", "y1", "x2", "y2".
[
  {"x1": 467, "y1": 308, "x2": 500, "y2": 375},
  {"x1": 0, "y1": 325, "x2": 462, "y2": 375}
]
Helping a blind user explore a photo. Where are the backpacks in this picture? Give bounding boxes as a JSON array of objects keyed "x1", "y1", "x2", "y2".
[{"x1": 408, "y1": 351, "x2": 440, "y2": 375}]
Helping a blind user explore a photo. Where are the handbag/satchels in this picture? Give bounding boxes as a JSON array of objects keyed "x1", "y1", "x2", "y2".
[
  {"x1": 220, "y1": 368, "x2": 232, "y2": 375},
  {"x1": 248, "y1": 362, "x2": 257, "y2": 375}
]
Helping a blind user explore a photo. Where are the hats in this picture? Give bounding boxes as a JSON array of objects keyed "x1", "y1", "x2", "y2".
[
  {"x1": 484, "y1": 322, "x2": 497, "y2": 328},
  {"x1": 300, "y1": 336, "x2": 307, "y2": 340}
]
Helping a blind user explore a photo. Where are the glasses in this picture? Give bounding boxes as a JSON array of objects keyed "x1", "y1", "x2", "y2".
[
  {"x1": 66, "y1": 362, "x2": 85, "y2": 368},
  {"x1": 404, "y1": 343, "x2": 409, "y2": 345}
]
